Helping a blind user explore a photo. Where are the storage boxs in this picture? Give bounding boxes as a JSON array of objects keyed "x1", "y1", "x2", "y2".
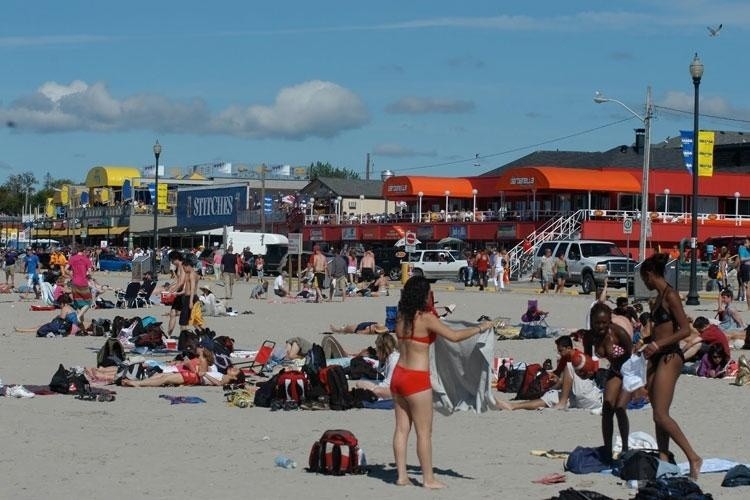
[{"x1": 160, "y1": 291, "x2": 176, "y2": 303}]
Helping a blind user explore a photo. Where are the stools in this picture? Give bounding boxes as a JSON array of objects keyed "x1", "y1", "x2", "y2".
[{"x1": 528, "y1": 311, "x2": 548, "y2": 327}]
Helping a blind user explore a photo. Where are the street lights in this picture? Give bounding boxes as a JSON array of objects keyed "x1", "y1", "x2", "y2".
[
  {"x1": 149, "y1": 137, "x2": 163, "y2": 281},
  {"x1": 592, "y1": 85, "x2": 659, "y2": 264},
  {"x1": 685, "y1": 51, "x2": 705, "y2": 310}
]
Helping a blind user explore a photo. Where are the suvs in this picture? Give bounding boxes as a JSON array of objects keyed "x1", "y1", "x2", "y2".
[
  {"x1": 533, "y1": 239, "x2": 637, "y2": 297},
  {"x1": 399, "y1": 249, "x2": 476, "y2": 284}
]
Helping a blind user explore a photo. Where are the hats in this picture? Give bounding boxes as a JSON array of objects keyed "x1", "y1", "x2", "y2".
[
  {"x1": 199, "y1": 285, "x2": 213, "y2": 292},
  {"x1": 26, "y1": 246, "x2": 34, "y2": 251}
]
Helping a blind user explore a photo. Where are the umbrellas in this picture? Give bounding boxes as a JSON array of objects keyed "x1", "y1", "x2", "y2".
[
  {"x1": 394, "y1": 236, "x2": 421, "y2": 276},
  {"x1": 436, "y1": 236, "x2": 464, "y2": 250}
]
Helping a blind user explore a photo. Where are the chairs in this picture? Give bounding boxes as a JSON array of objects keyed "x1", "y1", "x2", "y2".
[
  {"x1": 135, "y1": 281, "x2": 156, "y2": 308},
  {"x1": 115, "y1": 282, "x2": 140, "y2": 309},
  {"x1": 230, "y1": 340, "x2": 276, "y2": 376}
]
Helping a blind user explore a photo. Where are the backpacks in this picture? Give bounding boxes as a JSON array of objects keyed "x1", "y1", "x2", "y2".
[
  {"x1": 97, "y1": 336, "x2": 126, "y2": 367},
  {"x1": 111, "y1": 315, "x2": 161, "y2": 346},
  {"x1": 115, "y1": 362, "x2": 144, "y2": 387},
  {"x1": 254, "y1": 368, "x2": 286, "y2": 406},
  {"x1": 97, "y1": 298, "x2": 114, "y2": 309},
  {"x1": 320, "y1": 365, "x2": 353, "y2": 410},
  {"x1": 300, "y1": 364, "x2": 328, "y2": 399},
  {"x1": 631, "y1": 477, "x2": 713, "y2": 500},
  {"x1": 708, "y1": 260, "x2": 719, "y2": 280}
]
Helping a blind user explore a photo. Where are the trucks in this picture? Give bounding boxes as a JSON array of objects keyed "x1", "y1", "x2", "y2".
[{"x1": 205, "y1": 231, "x2": 289, "y2": 278}]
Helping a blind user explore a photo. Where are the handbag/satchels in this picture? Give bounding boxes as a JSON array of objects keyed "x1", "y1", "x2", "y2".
[{"x1": 564, "y1": 259, "x2": 568, "y2": 271}]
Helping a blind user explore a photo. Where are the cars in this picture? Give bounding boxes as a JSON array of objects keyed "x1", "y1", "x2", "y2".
[
  {"x1": 30, "y1": 252, "x2": 60, "y2": 273},
  {"x1": 354, "y1": 247, "x2": 404, "y2": 282},
  {"x1": 282, "y1": 251, "x2": 350, "y2": 279},
  {"x1": 94, "y1": 252, "x2": 132, "y2": 272}
]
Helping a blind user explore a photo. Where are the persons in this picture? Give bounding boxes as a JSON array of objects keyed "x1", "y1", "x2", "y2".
[
  {"x1": 133, "y1": 271, "x2": 156, "y2": 308},
  {"x1": 670, "y1": 240, "x2": 738, "y2": 254},
  {"x1": 55, "y1": 249, "x2": 65, "y2": 275},
  {"x1": 3, "y1": 247, "x2": 18, "y2": 291},
  {"x1": 84, "y1": 366, "x2": 118, "y2": 384},
  {"x1": 161, "y1": 340, "x2": 214, "y2": 373},
  {"x1": 146, "y1": 245, "x2": 170, "y2": 257},
  {"x1": 94, "y1": 240, "x2": 119, "y2": 253},
  {"x1": 539, "y1": 248, "x2": 556, "y2": 295},
  {"x1": 243, "y1": 247, "x2": 253, "y2": 282},
  {"x1": 58, "y1": 295, "x2": 78, "y2": 335},
  {"x1": 251, "y1": 279, "x2": 269, "y2": 300},
  {"x1": 199, "y1": 285, "x2": 216, "y2": 317},
  {"x1": 708, "y1": 246, "x2": 729, "y2": 312},
  {"x1": 731, "y1": 255, "x2": 746, "y2": 300},
  {"x1": 321, "y1": 336, "x2": 356, "y2": 359},
  {"x1": 716, "y1": 290, "x2": 743, "y2": 330},
  {"x1": 502, "y1": 249, "x2": 509, "y2": 273},
  {"x1": 390, "y1": 277, "x2": 497, "y2": 488},
  {"x1": 330, "y1": 322, "x2": 389, "y2": 334},
  {"x1": 586, "y1": 275, "x2": 652, "y2": 329},
  {"x1": 235, "y1": 253, "x2": 242, "y2": 280},
  {"x1": 278, "y1": 337, "x2": 314, "y2": 360},
  {"x1": 327, "y1": 248, "x2": 348, "y2": 302},
  {"x1": 475, "y1": 247, "x2": 491, "y2": 290},
  {"x1": 221, "y1": 246, "x2": 237, "y2": 298},
  {"x1": 41, "y1": 274, "x2": 73, "y2": 305},
  {"x1": 134, "y1": 247, "x2": 144, "y2": 258},
  {"x1": 0, "y1": 243, "x2": 37, "y2": 254},
  {"x1": 298, "y1": 263, "x2": 313, "y2": 293},
  {"x1": 167, "y1": 251, "x2": 185, "y2": 335},
  {"x1": 51, "y1": 247, "x2": 59, "y2": 271},
  {"x1": 121, "y1": 368, "x2": 244, "y2": 387},
  {"x1": 724, "y1": 330, "x2": 747, "y2": 341},
  {"x1": 214, "y1": 250, "x2": 222, "y2": 280},
  {"x1": 274, "y1": 271, "x2": 288, "y2": 296},
  {"x1": 19, "y1": 247, "x2": 40, "y2": 299},
  {"x1": 172, "y1": 259, "x2": 199, "y2": 331},
  {"x1": 631, "y1": 253, "x2": 702, "y2": 479},
  {"x1": 547, "y1": 336, "x2": 583, "y2": 375},
  {"x1": 494, "y1": 363, "x2": 650, "y2": 410},
  {"x1": 555, "y1": 251, "x2": 569, "y2": 295},
  {"x1": 194, "y1": 244, "x2": 213, "y2": 258},
  {"x1": 356, "y1": 335, "x2": 402, "y2": 397},
  {"x1": 522, "y1": 237, "x2": 531, "y2": 270},
  {"x1": 371, "y1": 271, "x2": 390, "y2": 297},
  {"x1": 202, "y1": 257, "x2": 212, "y2": 280},
  {"x1": 739, "y1": 238, "x2": 750, "y2": 300},
  {"x1": 255, "y1": 254, "x2": 264, "y2": 283},
  {"x1": 584, "y1": 303, "x2": 632, "y2": 467},
  {"x1": 65, "y1": 244, "x2": 92, "y2": 322},
  {"x1": 466, "y1": 248, "x2": 478, "y2": 285},
  {"x1": 609, "y1": 206, "x2": 644, "y2": 223},
  {"x1": 114, "y1": 243, "x2": 133, "y2": 257},
  {"x1": 364, "y1": 247, "x2": 374, "y2": 256},
  {"x1": 326, "y1": 347, "x2": 377, "y2": 366},
  {"x1": 312, "y1": 245, "x2": 327, "y2": 302},
  {"x1": 348, "y1": 250, "x2": 357, "y2": 283},
  {"x1": 680, "y1": 317, "x2": 730, "y2": 360},
  {"x1": 681, "y1": 344, "x2": 730, "y2": 378},
  {"x1": 77, "y1": 197, "x2": 146, "y2": 208},
  {"x1": 304, "y1": 208, "x2": 526, "y2": 222},
  {"x1": 493, "y1": 248, "x2": 509, "y2": 293},
  {"x1": 360, "y1": 251, "x2": 375, "y2": 281}
]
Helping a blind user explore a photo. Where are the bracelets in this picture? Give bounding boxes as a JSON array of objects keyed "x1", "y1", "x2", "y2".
[
  {"x1": 475, "y1": 325, "x2": 483, "y2": 332},
  {"x1": 652, "y1": 342, "x2": 661, "y2": 352}
]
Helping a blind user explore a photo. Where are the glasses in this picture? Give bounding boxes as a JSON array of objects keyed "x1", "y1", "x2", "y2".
[
  {"x1": 546, "y1": 250, "x2": 551, "y2": 253},
  {"x1": 713, "y1": 355, "x2": 722, "y2": 359}
]
